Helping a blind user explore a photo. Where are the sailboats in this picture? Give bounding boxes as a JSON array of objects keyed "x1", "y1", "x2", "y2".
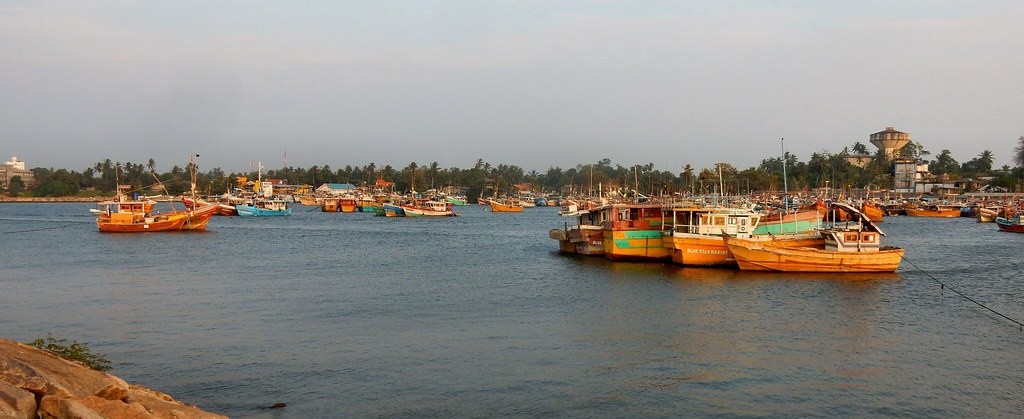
[
  {"x1": 563, "y1": 137, "x2": 861, "y2": 213},
  {"x1": 180, "y1": 154, "x2": 294, "y2": 218}
]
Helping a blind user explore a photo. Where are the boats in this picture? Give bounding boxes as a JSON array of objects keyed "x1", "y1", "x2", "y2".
[
  {"x1": 548, "y1": 198, "x2": 605, "y2": 256},
  {"x1": 300, "y1": 188, "x2": 469, "y2": 216},
  {"x1": 832, "y1": 187, "x2": 1023, "y2": 232},
  {"x1": 602, "y1": 200, "x2": 824, "y2": 259},
  {"x1": 90, "y1": 152, "x2": 219, "y2": 233},
  {"x1": 717, "y1": 206, "x2": 904, "y2": 275},
  {"x1": 661, "y1": 204, "x2": 760, "y2": 266},
  {"x1": 476, "y1": 184, "x2": 560, "y2": 212}
]
